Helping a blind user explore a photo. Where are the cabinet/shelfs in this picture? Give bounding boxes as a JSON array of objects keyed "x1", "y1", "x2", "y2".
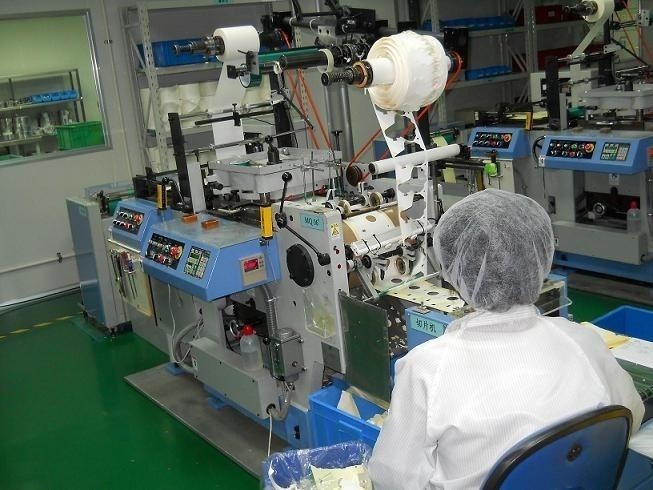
[
  {"x1": 117, "y1": 0, "x2": 313, "y2": 172},
  {"x1": 408, "y1": 0, "x2": 533, "y2": 126},
  {"x1": 531, "y1": 0, "x2": 615, "y2": 76},
  {"x1": 0, "y1": 68, "x2": 86, "y2": 156}
]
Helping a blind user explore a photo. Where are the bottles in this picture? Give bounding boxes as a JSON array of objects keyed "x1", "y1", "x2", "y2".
[
  {"x1": 239, "y1": 326, "x2": 264, "y2": 371},
  {"x1": 626, "y1": 202, "x2": 640, "y2": 235}
]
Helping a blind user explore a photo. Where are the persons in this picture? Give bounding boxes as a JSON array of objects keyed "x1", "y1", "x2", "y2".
[{"x1": 368, "y1": 190, "x2": 645, "y2": 489}]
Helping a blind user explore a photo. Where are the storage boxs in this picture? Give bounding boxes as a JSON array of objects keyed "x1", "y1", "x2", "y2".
[
  {"x1": 55, "y1": 121, "x2": 104, "y2": 150},
  {"x1": 308, "y1": 386, "x2": 381, "y2": 452},
  {"x1": 591, "y1": 304, "x2": 653, "y2": 343}
]
